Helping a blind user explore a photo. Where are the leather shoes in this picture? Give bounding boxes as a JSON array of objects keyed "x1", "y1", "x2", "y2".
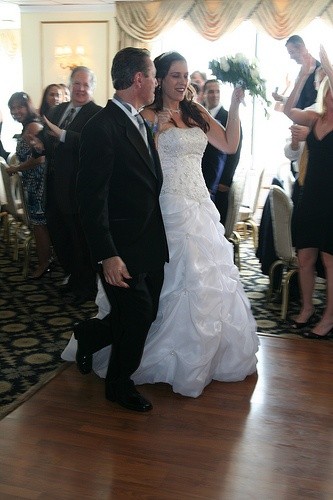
[
  {"x1": 114, "y1": 393, "x2": 153, "y2": 413},
  {"x1": 71, "y1": 322, "x2": 94, "y2": 375}
]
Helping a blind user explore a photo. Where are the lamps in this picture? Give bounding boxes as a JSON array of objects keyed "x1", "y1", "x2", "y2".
[{"x1": 54, "y1": 45, "x2": 83, "y2": 70}]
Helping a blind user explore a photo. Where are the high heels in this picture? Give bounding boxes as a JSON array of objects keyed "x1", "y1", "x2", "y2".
[
  {"x1": 302, "y1": 328, "x2": 333, "y2": 339},
  {"x1": 24, "y1": 257, "x2": 55, "y2": 281},
  {"x1": 291, "y1": 313, "x2": 317, "y2": 329}
]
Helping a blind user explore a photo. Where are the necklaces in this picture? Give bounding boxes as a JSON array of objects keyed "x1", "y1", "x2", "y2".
[{"x1": 162, "y1": 106, "x2": 183, "y2": 114}]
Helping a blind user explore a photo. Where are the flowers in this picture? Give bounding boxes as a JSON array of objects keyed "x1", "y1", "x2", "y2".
[
  {"x1": 146, "y1": 119, "x2": 158, "y2": 140},
  {"x1": 208, "y1": 52, "x2": 273, "y2": 119}
]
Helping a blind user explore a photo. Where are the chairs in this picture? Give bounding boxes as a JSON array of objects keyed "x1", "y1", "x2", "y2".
[
  {"x1": 222, "y1": 164, "x2": 298, "y2": 321},
  {"x1": 0, "y1": 149, "x2": 56, "y2": 282}
]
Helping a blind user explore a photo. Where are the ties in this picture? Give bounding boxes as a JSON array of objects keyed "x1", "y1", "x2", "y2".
[
  {"x1": 58, "y1": 108, "x2": 77, "y2": 131},
  {"x1": 135, "y1": 113, "x2": 147, "y2": 143}
]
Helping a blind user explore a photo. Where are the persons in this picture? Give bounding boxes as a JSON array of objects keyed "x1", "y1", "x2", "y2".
[
  {"x1": 284, "y1": 43, "x2": 333, "y2": 341},
  {"x1": 270, "y1": 33, "x2": 328, "y2": 276},
  {"x1": 136, "y1": 50, "x2": 246, "y2": 380},
  {"x1": 75, "y1": 46, "x2": 170, "y2": 413},
  {"x1": 0, "y1": 83, "x2": 72, "y2": 279},
  {"x1": 21, "y1": 66, "x2": 104, "y2": 308},
  {"x1": 186, "y1": 69, "x2": 243, "y2": 228}
]
